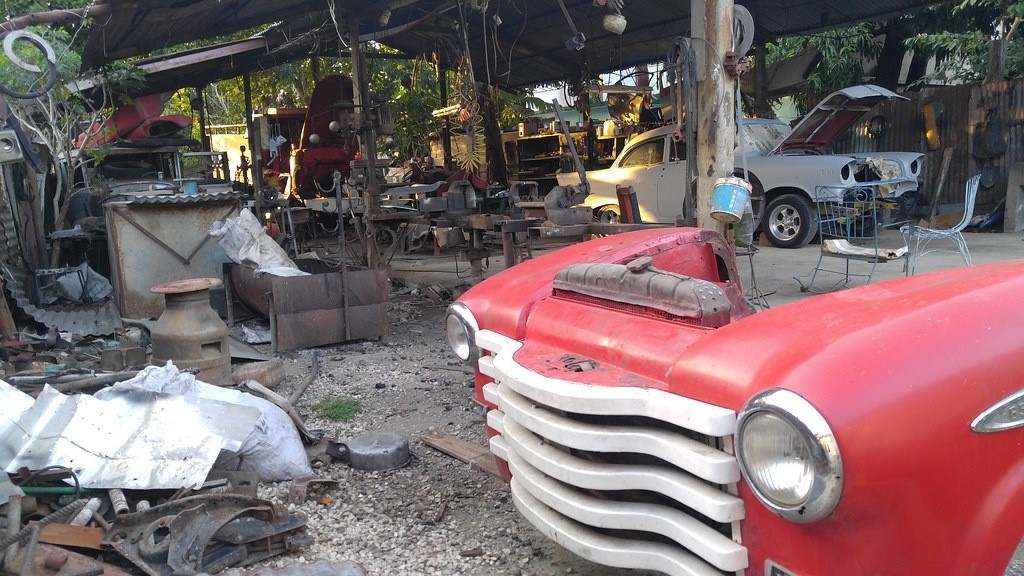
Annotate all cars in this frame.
[444,224,1024,576]
[556,84,925,250]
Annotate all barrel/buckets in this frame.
[709,177,752,224]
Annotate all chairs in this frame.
[900,173,982,276]
[795,186,912,296]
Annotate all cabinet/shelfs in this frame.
[503,131,589,197]
[595,134,629,167]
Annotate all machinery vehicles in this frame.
[259,78,362,202]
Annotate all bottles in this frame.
[603,120,614,137]
